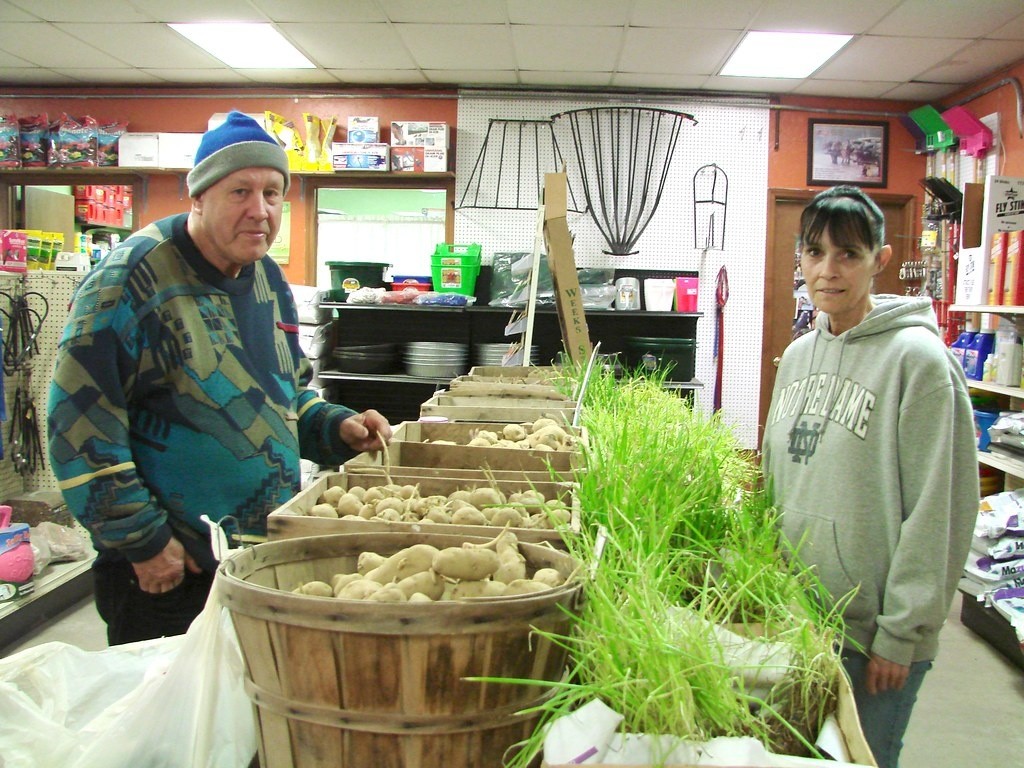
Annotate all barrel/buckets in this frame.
[325,261,393,301]
[214,532,591,768]
[975,410,999,453]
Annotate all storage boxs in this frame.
[158,133,204,168]
[391,121,450,149]
[119,132,158,166]
[332,142,390,171]
[347,116,381,143]
[391,147,449,172]
[208,113,266,132]
[265,366,590,550]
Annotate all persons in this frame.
[857,140,866,167]
[760,185,980,768]
[840,146,849,164]
[48,109,393,647]
[861,140,881,177]
[825,135,843,164]
[843,139,855,165]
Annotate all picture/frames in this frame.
[806,117,889,188]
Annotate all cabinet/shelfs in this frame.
[316,302,704,424]
[946,303,1024,479]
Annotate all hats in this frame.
[187,112,290,198]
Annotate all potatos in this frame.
[287,417,579,603]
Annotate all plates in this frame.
[334,342,538,377]
